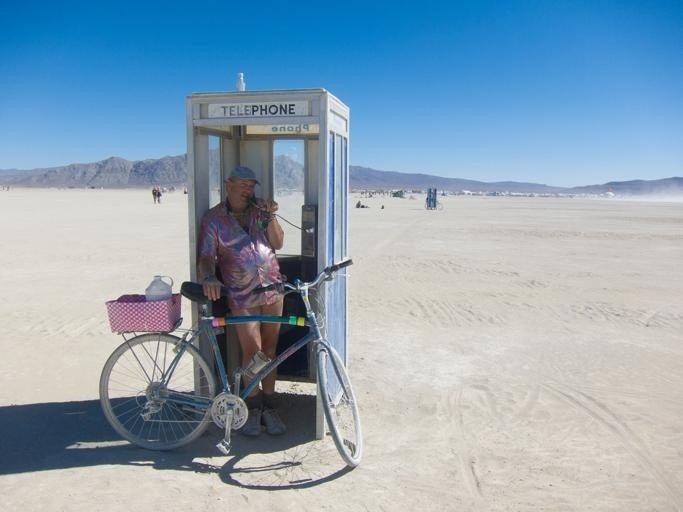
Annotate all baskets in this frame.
[106,294,181,332]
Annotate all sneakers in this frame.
[243,404,263,436]
[262,407,287,435]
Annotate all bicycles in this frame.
[99,259,361,469]
[425,200,443,209]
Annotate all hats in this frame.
[231,167,261,186]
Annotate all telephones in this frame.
[250,192,267,209]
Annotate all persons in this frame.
[196,165,288,437]
[151,186,156,204]
[156,186,162,204]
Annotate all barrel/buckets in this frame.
[145,274,174,301]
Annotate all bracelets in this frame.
[200,273,218,282]
